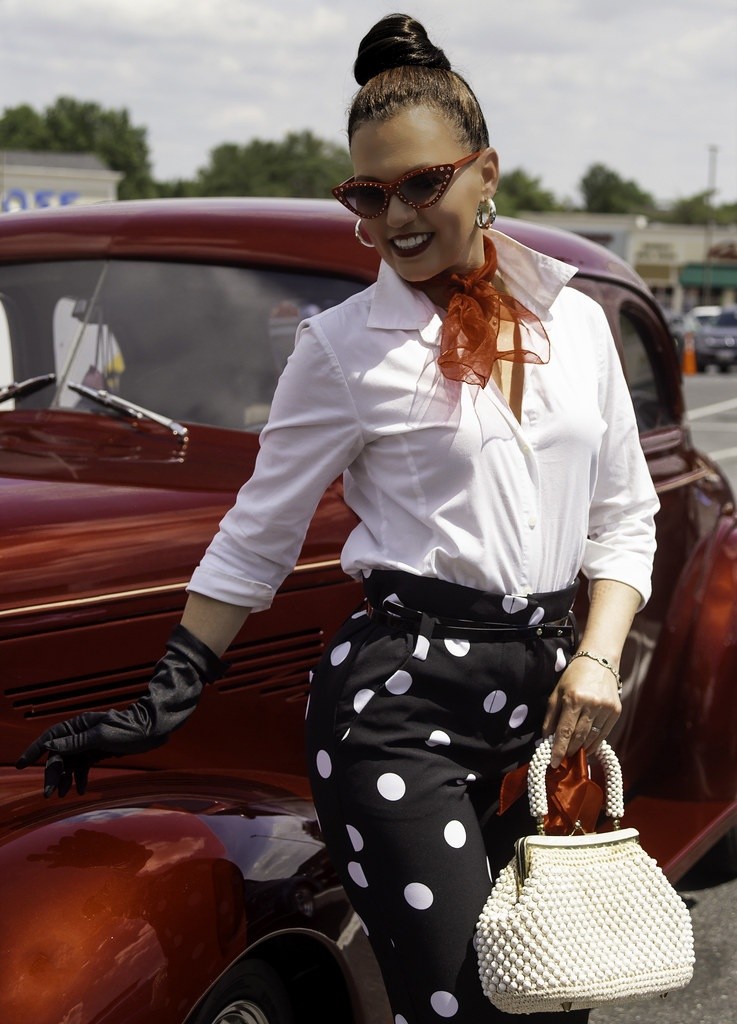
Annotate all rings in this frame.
[592,726,600,733]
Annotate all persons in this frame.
[15,14,661,1024]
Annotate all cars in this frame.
[681,305,737,369]
[1,197,737,1021]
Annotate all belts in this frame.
[362,593,577,639]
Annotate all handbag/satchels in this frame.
[475,729,696,1014]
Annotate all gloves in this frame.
[14,623,224,801]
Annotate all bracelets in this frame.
[569,651,623,694]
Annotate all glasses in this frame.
[330,149,491,220]
